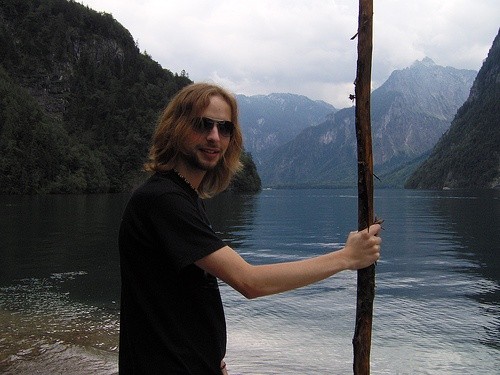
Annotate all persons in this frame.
[117,83,384,375]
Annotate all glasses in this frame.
[192,117,235,137]
